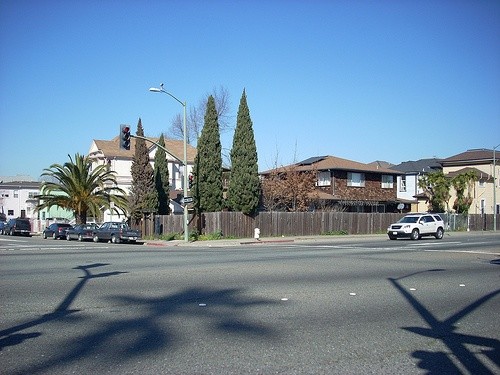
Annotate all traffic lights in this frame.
[120,124,131,150]
[188,174,193,189]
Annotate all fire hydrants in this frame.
[253,227,260,241]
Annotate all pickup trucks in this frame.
[92,222,142,243]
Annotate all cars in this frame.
[0,220,7,234]
[42,223,73,240]
[65,223,100,241]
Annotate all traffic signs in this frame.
[183,197,193,203]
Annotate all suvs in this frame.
[386,212,445,240]
[2,217,32,236]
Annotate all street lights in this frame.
[148,87,191,243]
